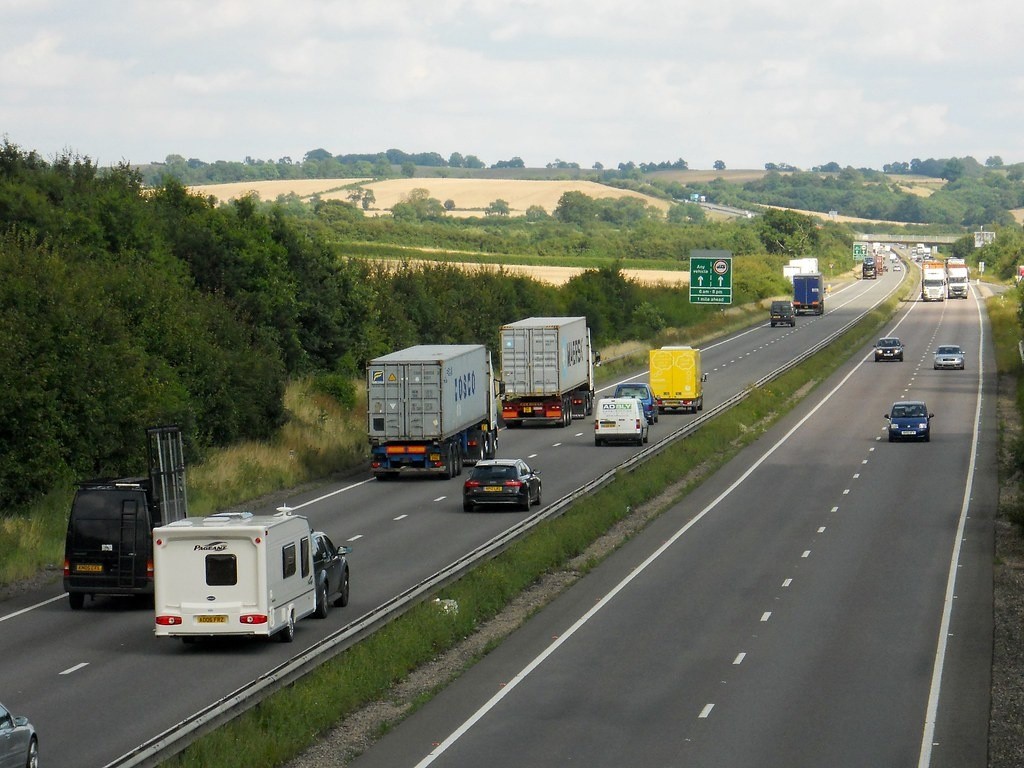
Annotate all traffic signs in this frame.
[689,257,733,305]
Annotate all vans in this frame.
[770,301,795,327]
[595,395,650,447]
[61,477,160,609]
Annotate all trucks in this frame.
[792,272,826,316]
[945,257,969,299]
[875,256,882,275]
[649,346,707,414]
[916,244,924,255]
[153,511,317,644]
[498,315,602,429]
[863,260,877,280]
[365,343,507,482]
[921,260,946,302]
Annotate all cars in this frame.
[879,243,936,272]
[932,345,966,370]
[462,459,543,512]
[311,530,351,617]
[873,337,906,362]
[614,383,661,425]
[883,401,934,442]
[0,705,39,768]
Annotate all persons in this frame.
[881,340,886,347]
[895,409,904,417]
[891,340,897,347]
[912,407,922,417]
